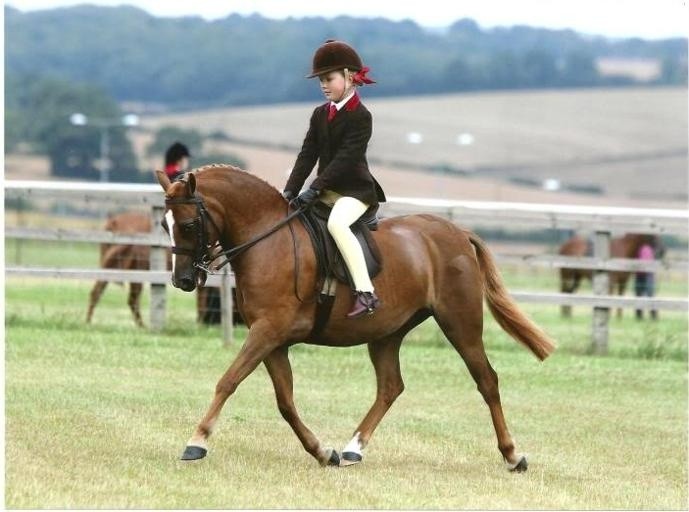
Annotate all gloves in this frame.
[282,191,294,201]
[298,190,318,208]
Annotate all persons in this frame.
[631,234,657,319]
[285,39,387,319]
[165,142,190,182]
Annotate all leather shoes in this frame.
[345,293,380,320]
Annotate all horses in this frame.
[154,162,559,474]
[558,234,666,319]
[85,207,210,328]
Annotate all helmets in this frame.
[165,143,192,164]
[304,39,363,79]
